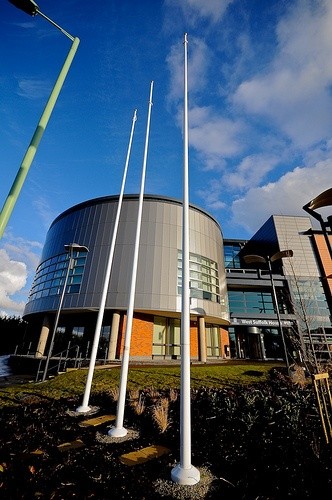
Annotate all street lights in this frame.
[0,0,79,246]
